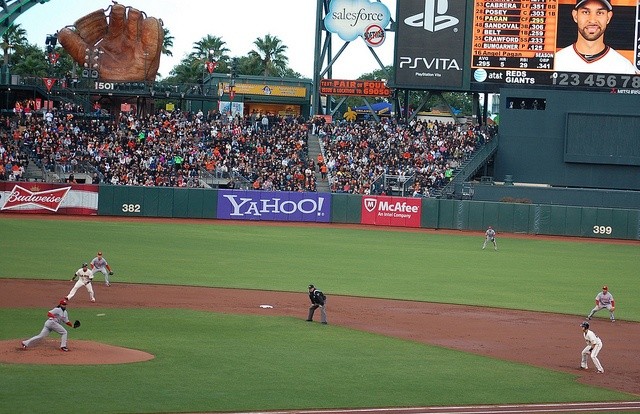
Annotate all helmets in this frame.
[580,322,589,329]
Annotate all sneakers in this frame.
[306,320,313,321]
[581,367,588,369]
[586,317,591,320]
[322,322,327,324]
[61,347,69,351]
[610,319,615,322]
[106,283,109,287]
[21,342,26,349]
[595,370,601,373]
[91,298,95,302]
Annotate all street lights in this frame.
[83,48,104,112]
[225,57,239,118]
[143,52,149,94]
[209,47,214,92]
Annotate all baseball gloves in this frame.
[109,272,113,275]
[74,320,80,328]
[610,307,615,312]
[491,239,494,242]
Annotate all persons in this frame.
[319,163,326,181]
[305,284,328,324]
[72,74,78,88]
[586,285,616,322]
[90,251,112,287]
[21,298,74,352]
[325,146,476,198]
[333,118,498,147]
[483,226,497,250]
[579,321,605,374]
[64,263,95,303]
[317,153,324,173]
[1,100,318,192]
[317,116,332,152]
[65,71,72,87]
[554,0,635,74]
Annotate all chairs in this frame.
[460,187,475,200]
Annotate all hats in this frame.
[81,263,88,268]
[574,0,613,10]
[60,300,68,305]
[308,285,314,289]
[603,286,608,290]
[98,252,102,255]
